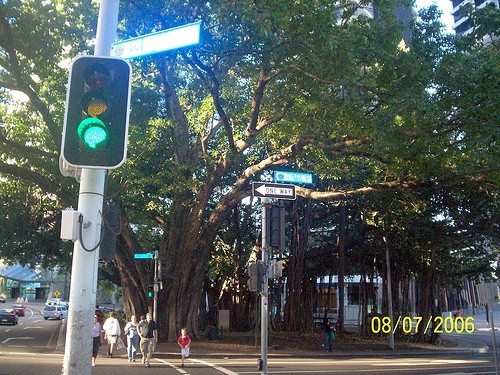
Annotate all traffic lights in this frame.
[147,286,154,299]
[248,263,262,292]
[61,57,132,170]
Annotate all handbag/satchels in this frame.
[116,337,126,350]
[331,332,335,341]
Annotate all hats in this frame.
[94,315,97,317]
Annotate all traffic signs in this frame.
[251,180,296,200]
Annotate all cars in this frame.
[11,304,26,316]
[96,305,113,312]
[0,295,6,303]
[0,307,19,325]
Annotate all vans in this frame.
[43,306,68,320]
[46,301,68,306]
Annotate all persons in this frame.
[177,328,191,359]
[324,319,333,353]
[137,313,161,367]
[455,308,461,322]
[91,315,101,367]
[124,316,140,363]
[103,312,121,355]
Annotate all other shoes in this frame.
[142,358,146,364]
[92,364,95,367]
[128,358,132,362]
[110,353,112,358]
[181,364,184,367]
[106,352,110,358]
[145,361,150,368]
[133,359,135,362]
